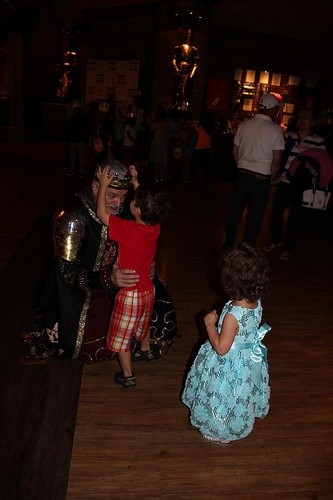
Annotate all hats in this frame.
[258,93,284,109]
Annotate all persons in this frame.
[177,242,273,447]
[9,158,178,365]
[92,162,161,390]
[319,112,333,245]
[273,108,327,263]
[218,93,286,253]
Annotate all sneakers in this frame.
[114,371,137,389]
[131,349,155,363]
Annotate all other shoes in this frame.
[263,240,281,251]
[279,252,289,260]
